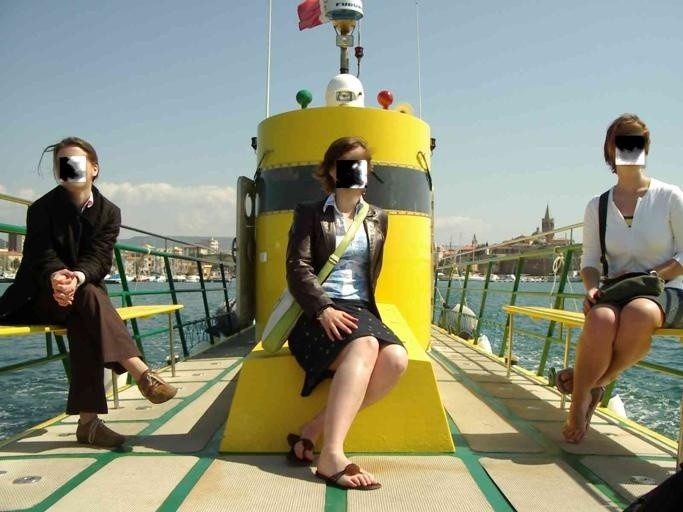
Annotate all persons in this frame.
[555,113,682,444]
[0,136,178,451]
[285,134,409,491]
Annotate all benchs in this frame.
[0,304,184,409]
[502,304,683,474]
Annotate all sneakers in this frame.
[138,370,177,405]
[75,419,124,449]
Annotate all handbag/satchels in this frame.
[589,272,664,305]
[260,289,304,354]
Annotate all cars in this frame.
[103,271,199,284]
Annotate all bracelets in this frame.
[317,306,333,321]
[647,267,657,277]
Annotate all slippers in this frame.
[285,435,315,466]
[315,464,381,491]
[563,386,602,442]
[556,368,573,395]
